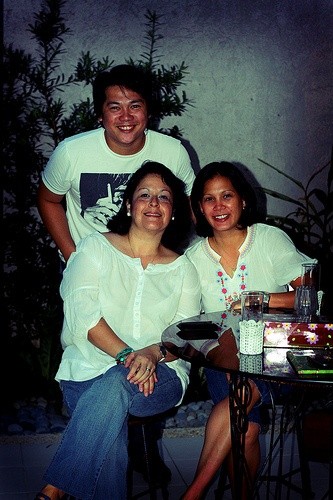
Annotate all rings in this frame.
[145,367,151,371]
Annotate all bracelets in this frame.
[114,347,136,363]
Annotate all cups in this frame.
[242,291,265,323]
[301,264,321,291]
[294,286,318,323]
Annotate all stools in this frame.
[128,415,168,500]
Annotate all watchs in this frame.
[153,343,167,363]
[262,291,271,307]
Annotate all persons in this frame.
[37,64,196,263]
[181,161,317,500]
[33,163,202,500]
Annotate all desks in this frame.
[161,306,333,500]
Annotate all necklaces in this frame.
[127,234,159,263]
